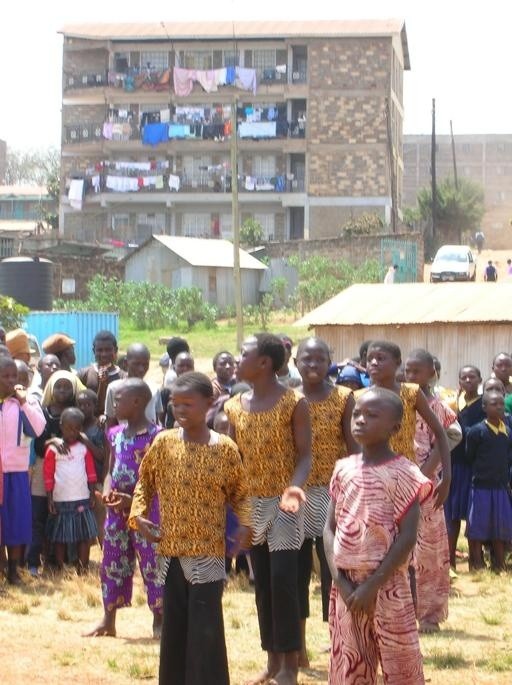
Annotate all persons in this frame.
[475,229,487,255]
[484,260,498,282]
[384,264,399,284]
[507,258,512,275]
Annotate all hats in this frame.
[5,329,36,358]
[42,334,76,354]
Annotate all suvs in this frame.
[429,244,477,283]
